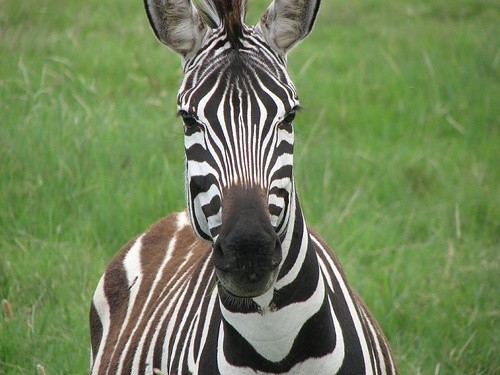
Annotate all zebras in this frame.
[90,0,397,375]
